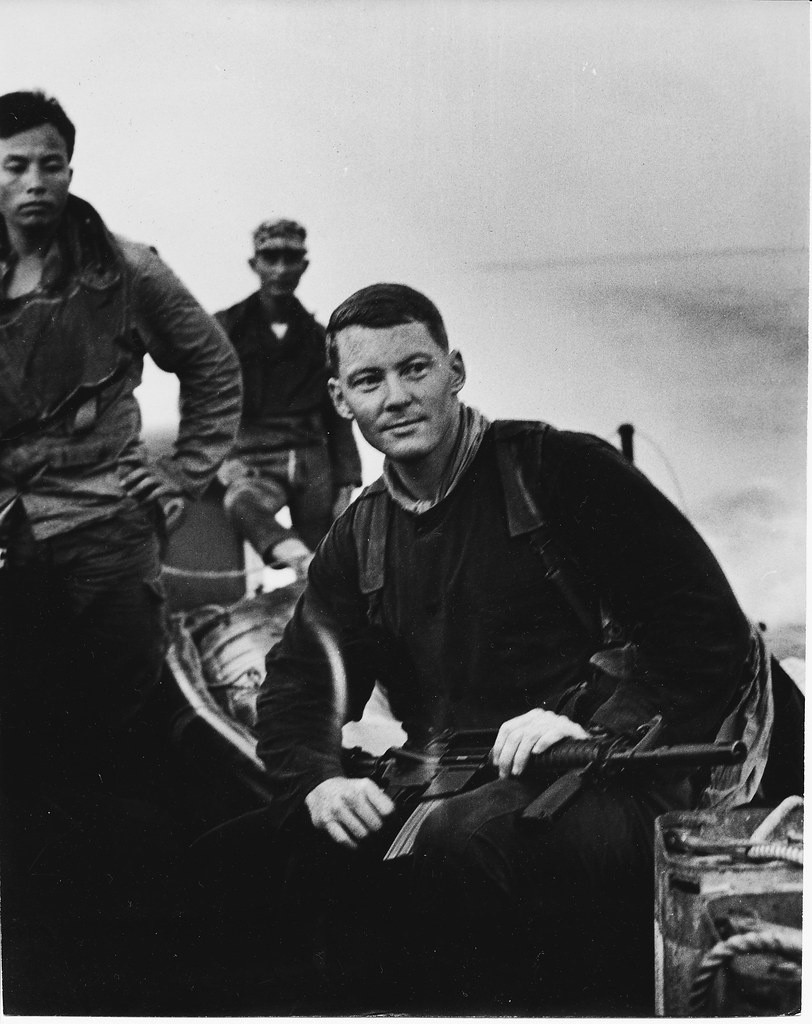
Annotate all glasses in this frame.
[258,247,305,265]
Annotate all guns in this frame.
[337,682,744,864]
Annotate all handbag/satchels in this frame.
[521,627,794,871]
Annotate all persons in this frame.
[256,282,774,886]
[0,89,363,727]
